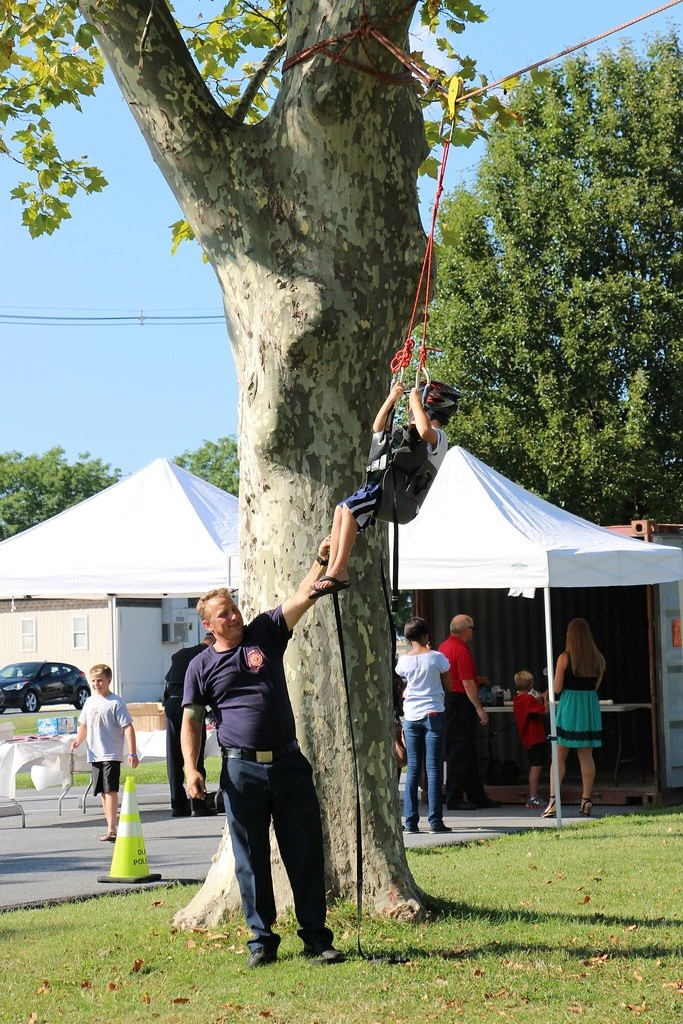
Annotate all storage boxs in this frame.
[37,715,77,736]
[124,702,165,730]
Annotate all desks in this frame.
[472,703,653,788]
[0,733,93,827]
[78,728,226,814]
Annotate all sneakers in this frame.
[526,796,548,808]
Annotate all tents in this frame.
[1,456,239,698]
[381,443,683,827]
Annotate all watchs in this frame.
[316,555,329,566]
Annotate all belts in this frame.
[222,739,300,763]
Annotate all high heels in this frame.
[578,797,592,818]
[542,796,557,818]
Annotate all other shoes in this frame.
[467,799,502,808]
[447,799,475,811]
[248,949,277,970]
[405,827,419,833]
[172,804,218,818]
[303,944,345,965]
[430,826,453,833]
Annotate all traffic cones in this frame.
[97,776,163,884]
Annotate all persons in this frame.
[512,668,550,809]
[541,618,605,819]
[395,617,451,834]
[163,632,217,818]
[309,378,463,603]
[395,638,408,832]
[437,614,503,810]
[71,664,140,842]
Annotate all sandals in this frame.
[101,832,117,841]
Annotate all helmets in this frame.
[403,380,461,426]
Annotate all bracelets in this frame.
[128,753,137,757]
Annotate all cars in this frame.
[0,661,92,714]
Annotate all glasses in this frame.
[468,626,474,631]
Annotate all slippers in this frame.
[312,574,351,590]
[308,588,334,599]
